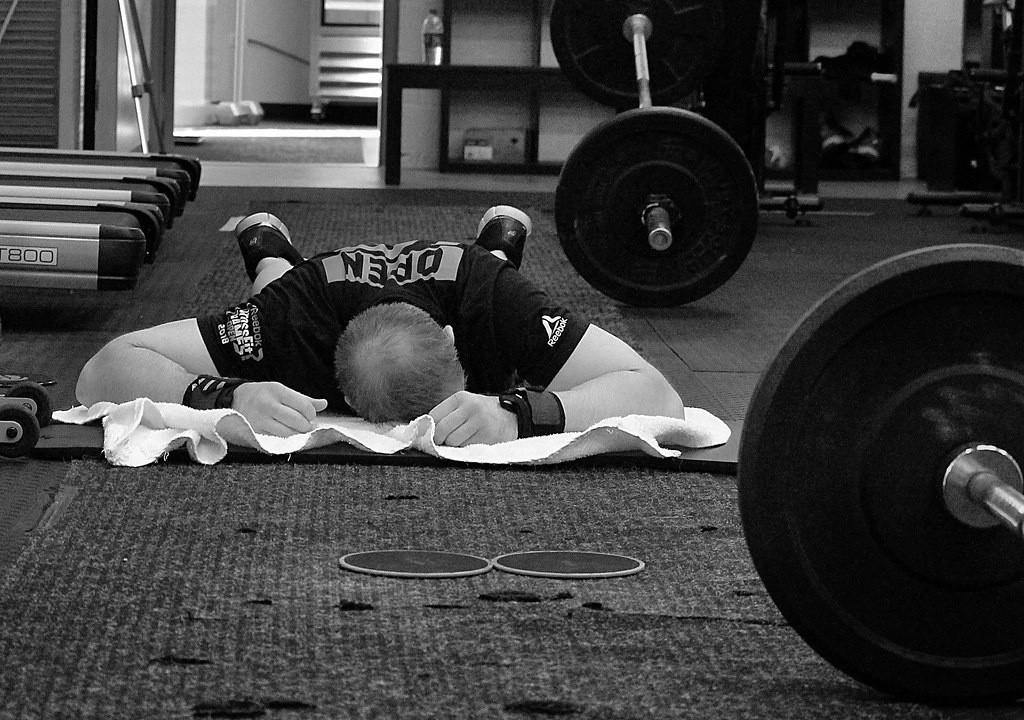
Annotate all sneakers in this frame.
[235,212,305,285]
[474,205,532,272]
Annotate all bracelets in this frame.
[499,386,566,441]
[181,372,250,411]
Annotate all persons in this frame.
[76,204,687,447]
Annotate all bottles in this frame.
[421,9,444,65]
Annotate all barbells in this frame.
[544,0,767,314]
[724,228,1024,715]
